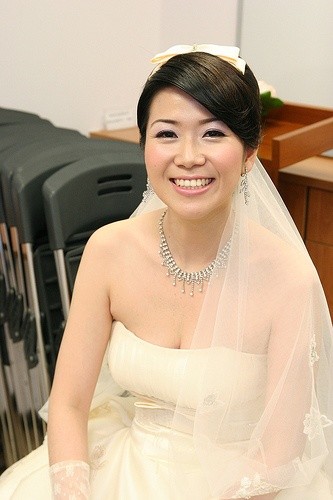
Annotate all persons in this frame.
[1,43,333,499]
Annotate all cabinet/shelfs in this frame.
[88,102,333,325]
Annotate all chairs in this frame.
[0,108,147,469]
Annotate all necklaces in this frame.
[157,206,239,300]
